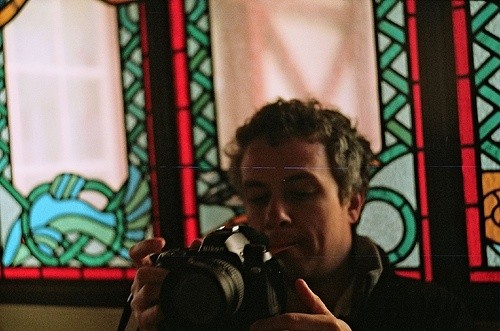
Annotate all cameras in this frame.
[150,226,290,331]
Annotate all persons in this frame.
[130,97,454,331]
